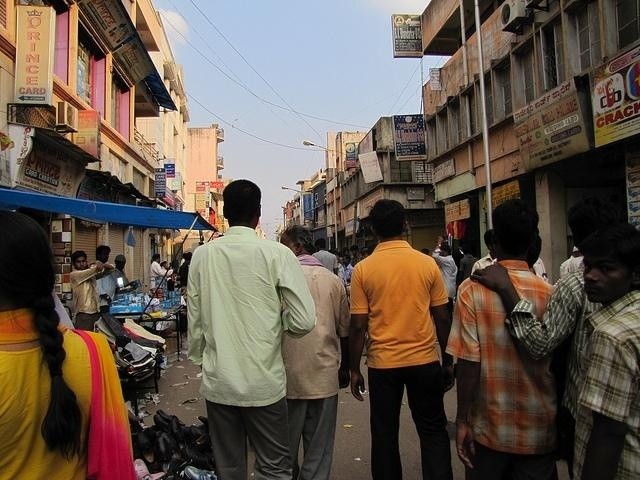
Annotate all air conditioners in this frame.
[56,102,78,132]
[498,0,526,29]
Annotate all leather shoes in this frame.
[130,409,219,480]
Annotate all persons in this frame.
[69,245,129,330]
[1,208,134,479]
[347,199,456,480]
[277,225,370,479]
[187,179,316,478]
[150,252,192,293]
[419,234,479,310]
[446,196,639,478]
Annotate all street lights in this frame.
[282,186,304,228]
[304,141,338,249]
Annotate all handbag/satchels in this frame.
[74,328,136,480]
